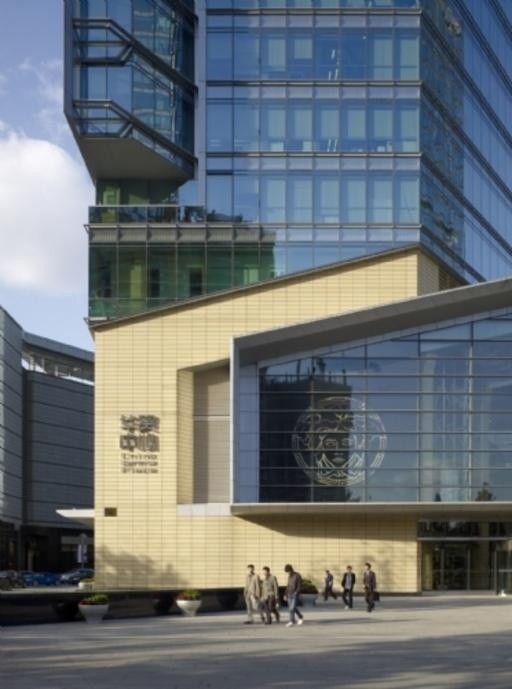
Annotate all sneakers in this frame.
[344,604,353,610]
[367,603,375,612]
[244,615,303,627]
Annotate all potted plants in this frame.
[77,578,95,591]
[297,578,319,607]
[78,594,111,623]
[174,591,201,616]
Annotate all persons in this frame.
[243,564,265,623]
[340,566,356,609]
[261,567,279,624]
[475,482,493,502]
[283,564,304,626]
[363,564,375,612]
[325,570,337,600]
